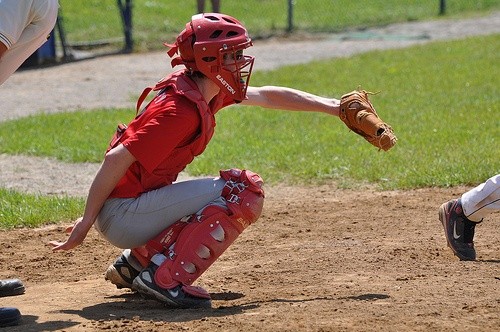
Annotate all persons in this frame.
[48,11,398,307]
[0,0,61,326]
[438,173,500,262]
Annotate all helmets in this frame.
[164,12,252,101]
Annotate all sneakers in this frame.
[105,249,152,296]
[133,254,212,309]
[437,199,483,261]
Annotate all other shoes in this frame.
[0,278,26,297]
[0,308,22,327]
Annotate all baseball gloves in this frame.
[337,85,400,152]
